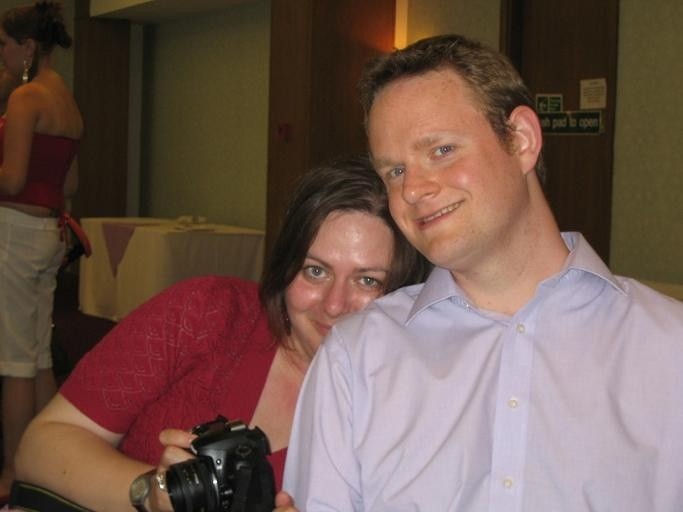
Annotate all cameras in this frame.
[165,414,275,512]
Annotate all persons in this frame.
[13,156,436,512]
[0,0,85,506]
[281,34,683,512]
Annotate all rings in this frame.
[155,473,166,490]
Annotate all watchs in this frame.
[129,469,157,512]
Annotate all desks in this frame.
[77,216,265,330]
